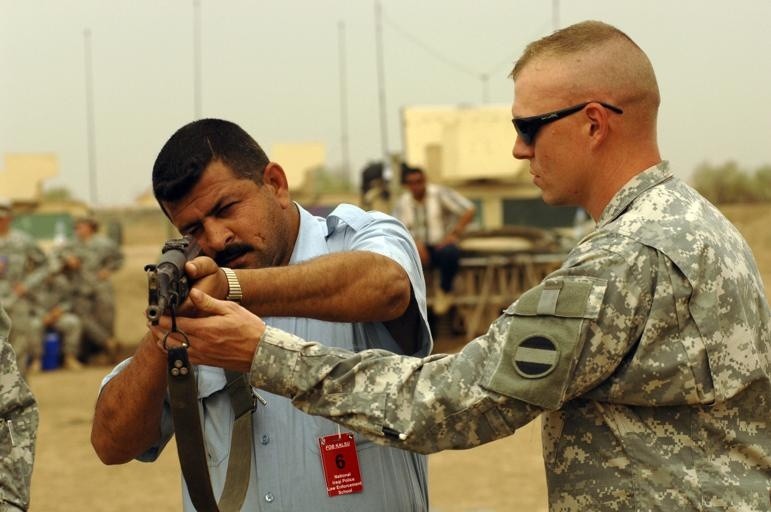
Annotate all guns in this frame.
[144,233,204,326]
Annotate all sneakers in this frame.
[433,289,451,313]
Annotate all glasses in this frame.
[513,102,623,146]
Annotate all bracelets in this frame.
[221,267,242,305]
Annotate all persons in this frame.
[392,169,476,315]
[0,304,39,512]
[91,119,434,512]
[0,219,125,370]
[144,21,770,512]
